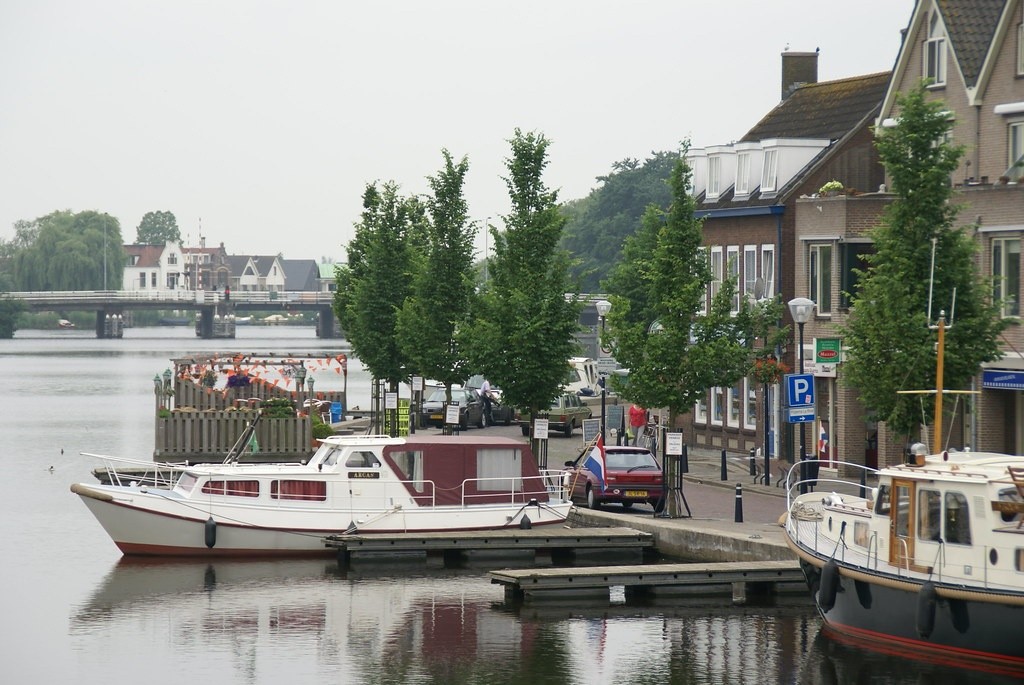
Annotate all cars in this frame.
[422,383,512,428]
[514,390,593,438]
[558,446,668,512]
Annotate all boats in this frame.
[778,238,1024,685]
[72,411,574,558]
[59,319,76,329]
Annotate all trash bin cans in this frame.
[806,455,819,486]
[331,401,342,423]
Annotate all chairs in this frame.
[305,399,333,425]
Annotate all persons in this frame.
[629,403,648,448]
[479,380,499,426]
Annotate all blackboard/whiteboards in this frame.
[582,419,601,444]
[607,406,623,431]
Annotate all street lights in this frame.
[787,298,817,495]
[596,299,611,446]
[103,212,108,292]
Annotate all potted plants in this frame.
[819,178,844,197]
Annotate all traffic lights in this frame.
[225,286,230,300]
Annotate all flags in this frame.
[249,432,259,454]
[582,433,609,494]
[818,421,829,453]
